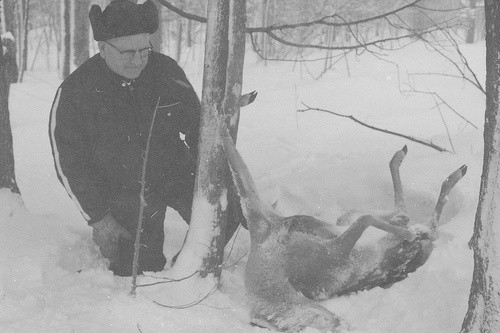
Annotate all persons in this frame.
[46,0,245,280]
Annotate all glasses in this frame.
[104,39,153,58]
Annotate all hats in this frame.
[90,0,158,41]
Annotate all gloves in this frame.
[92,214,131,260]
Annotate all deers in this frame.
[219,89,469,333]
